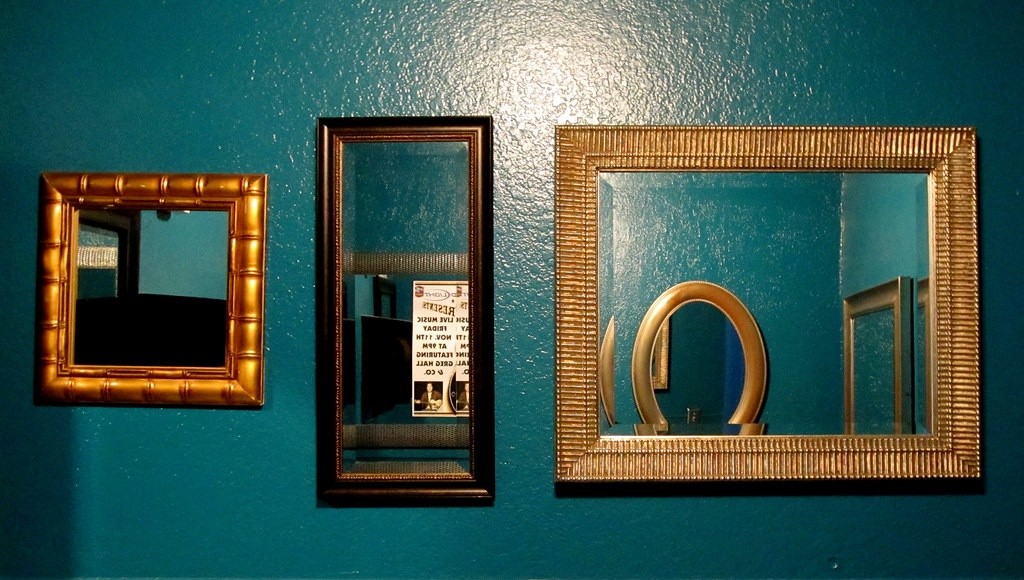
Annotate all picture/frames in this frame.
[651,317,670,391]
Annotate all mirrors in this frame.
[315,115,496,507]
[839,274,912,435]
[631,279,768,425]
[31,170,267,408]
[553,123,982,484]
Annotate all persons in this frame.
[421,383,442,411]
[458,382,469,410]
